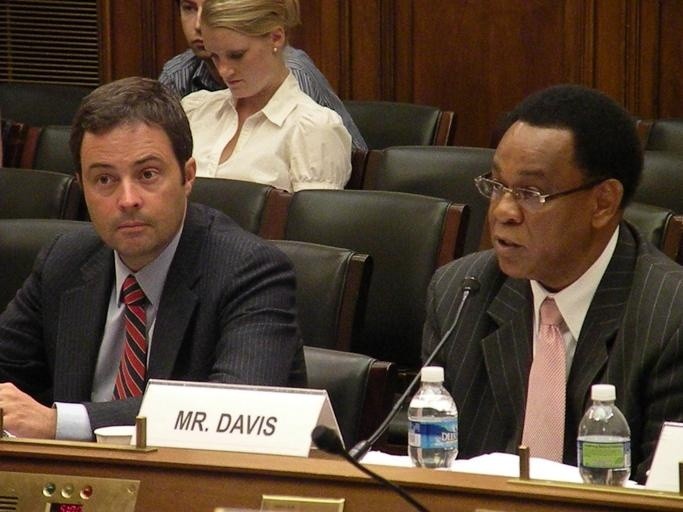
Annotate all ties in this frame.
[519,298,568,463]
[109,275,150,399]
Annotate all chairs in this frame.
[622,201,683,263]
[19,125,75,174]
[0,167,74,222]
[1,219,91,313]
[343,101,457,150]
[66,177,289,239]
[305,347,396,449]
[344,148,367,191]
[632,119,683,150]
[630,150,683,213]
[366,146,495,258]
[276,190,471,370]
[0,119,25,168]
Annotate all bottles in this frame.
[577,385,631,491]
[407,365,458,470]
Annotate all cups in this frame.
[93,425,135,445]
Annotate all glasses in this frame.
[473,169,611,216]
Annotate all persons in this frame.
[421,86,682,482]
[0,76,308,441]
[159,1,369,161]
[180,0,353,193]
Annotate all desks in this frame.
[0,433,683,512]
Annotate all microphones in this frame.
[350,275,481,462]
[310,425,432,512]
